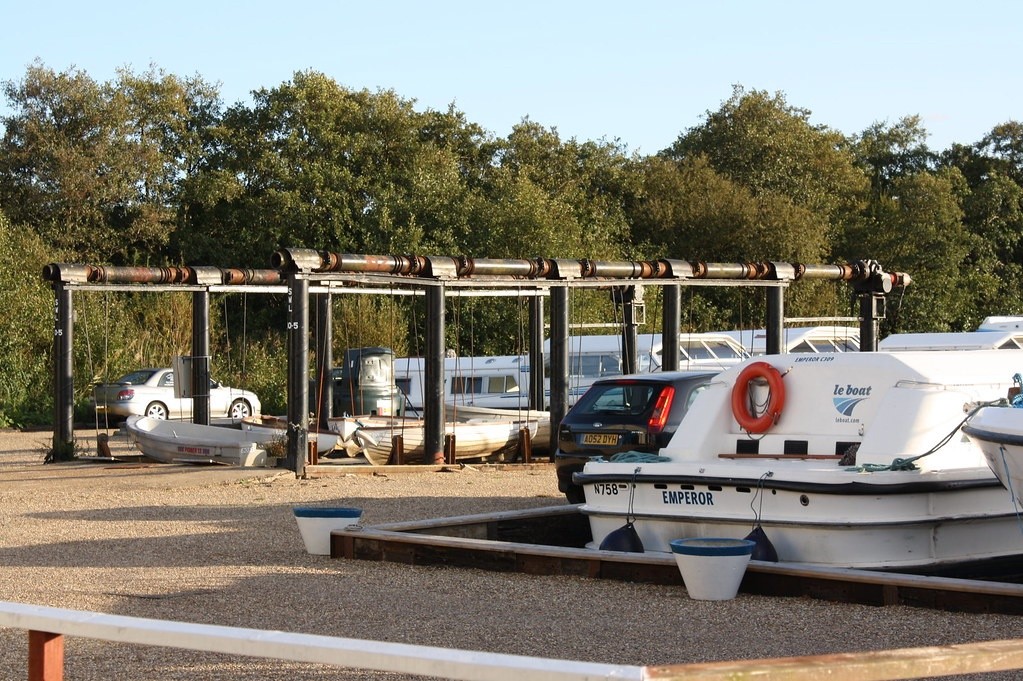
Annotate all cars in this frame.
[92,366,263,429]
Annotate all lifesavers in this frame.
[730,360,787,434]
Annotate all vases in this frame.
[292,508,364,555]
[670,536,758,601]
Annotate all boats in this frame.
[310,318,860,407]
[126,412,289,467]
[444,401,552,455]
[355,417,538,469]
[322,411,398,451]
[962,374,1023,515]
[570,347,1023,591]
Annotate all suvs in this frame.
[553,368,720,502]
